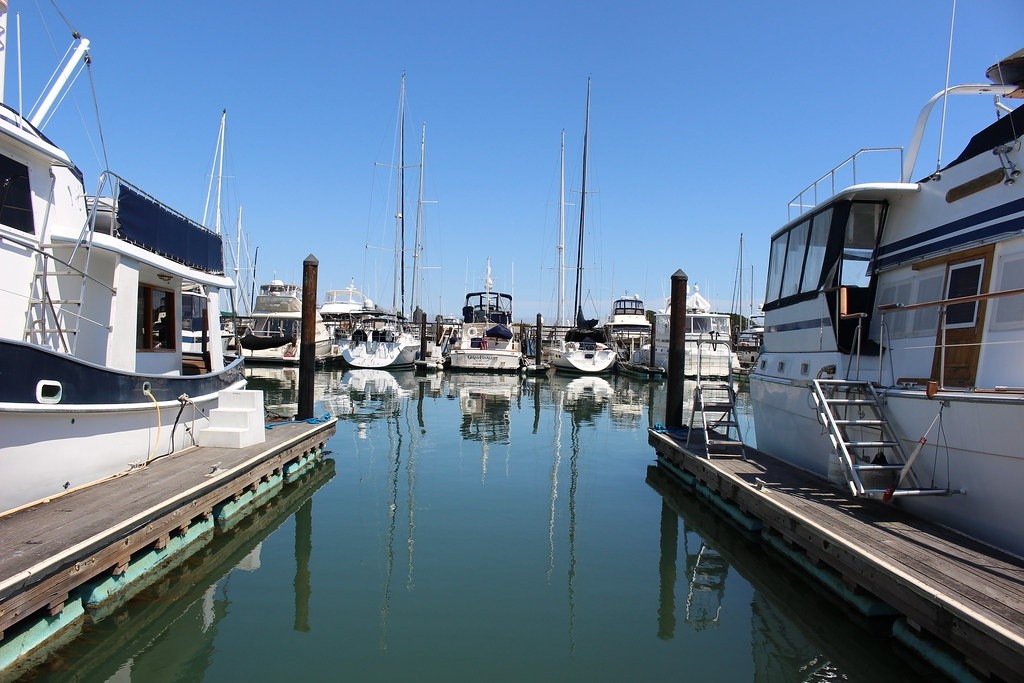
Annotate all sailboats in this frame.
[239,363,738,632]
[197,68,778,372]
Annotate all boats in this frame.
[748,45,1023,562]
[0,99,248,520]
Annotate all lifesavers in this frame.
[467,327,478,337]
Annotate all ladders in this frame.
[686,341,747,461]
[682,520,730,624]
